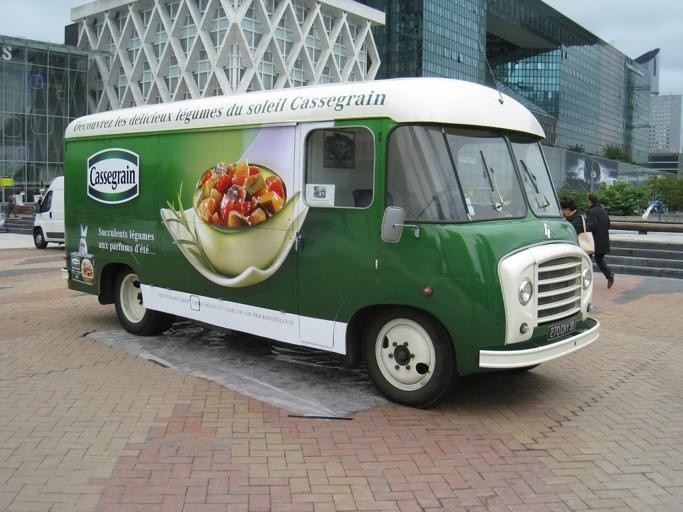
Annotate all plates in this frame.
[158,207,311,287]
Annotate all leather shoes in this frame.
[606,272,614,288]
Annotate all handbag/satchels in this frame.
[576,212,596,255]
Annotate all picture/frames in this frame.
[322,129,356,169]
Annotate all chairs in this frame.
[353,189,394,211]
[434,190,469,221]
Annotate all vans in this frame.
[34,176,65,249]
[64,77,601,407]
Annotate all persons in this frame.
[559,197,589,238]
[580,192,615,291]
